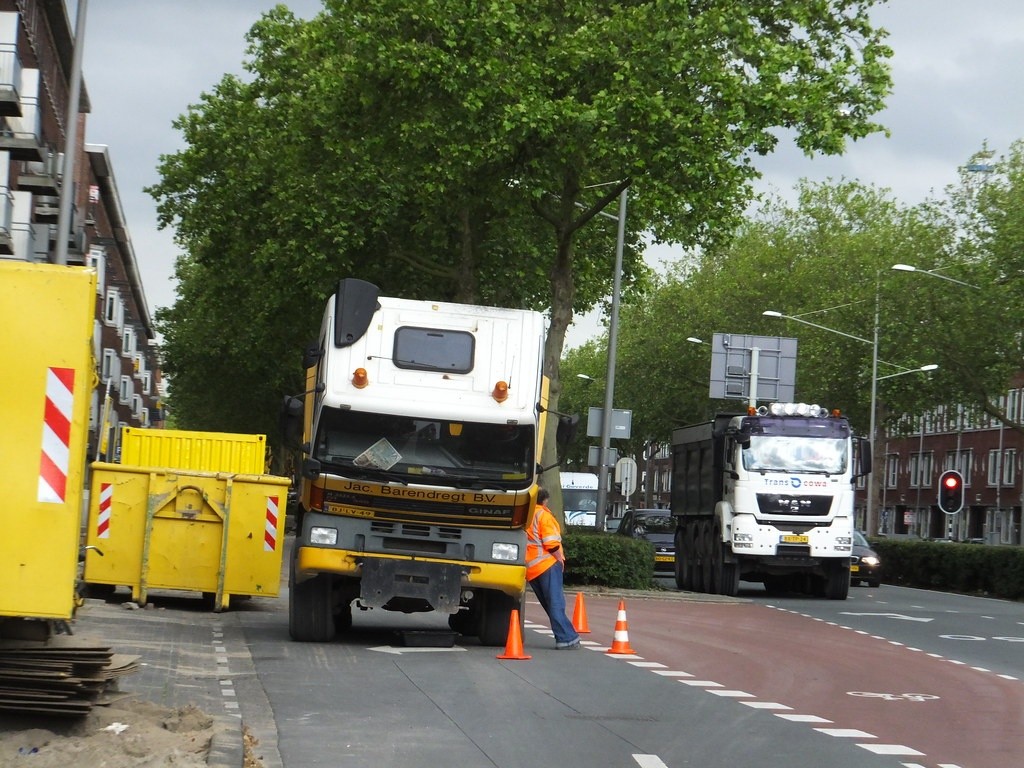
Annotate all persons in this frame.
[525,488,580,649]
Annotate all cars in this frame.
[614,508,678,572]
[850,530,881,587]
[964,537,990,546]
[923,537,955,543]
[606,517,623,533]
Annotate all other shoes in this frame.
[556,637,580,650]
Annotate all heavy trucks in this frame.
[671,403,872,601]
[282,278,580,649]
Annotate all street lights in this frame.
[763,270,939,538]
[495,175,627,531]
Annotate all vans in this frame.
[558,471,608,530]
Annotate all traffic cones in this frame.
[571,592,592,633]
[607,599,638,655]
[496,610,532,659]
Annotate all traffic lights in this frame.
[938,470,963,514]
